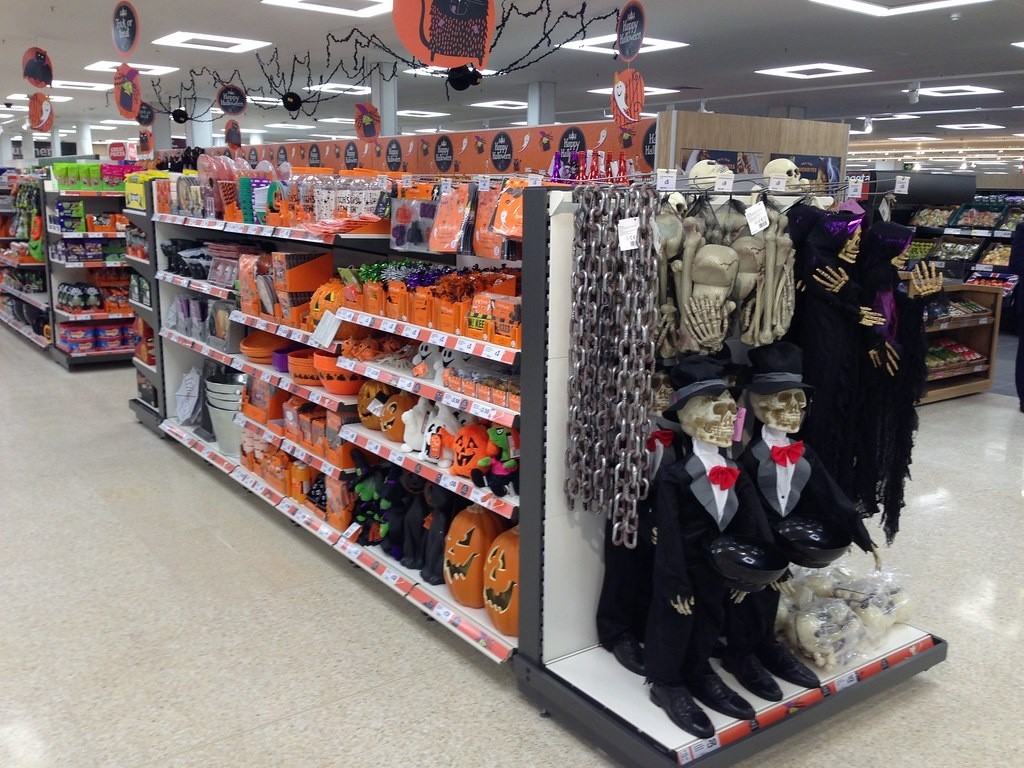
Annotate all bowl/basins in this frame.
[287,349,371,394]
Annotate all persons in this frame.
[1008,221,1024,413]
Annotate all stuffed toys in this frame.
[346,450,457,585]
[400,396,521,497]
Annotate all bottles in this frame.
[289,167,392,220]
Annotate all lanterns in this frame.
[341,334,421,361]
[357,379,421,443]
[310,272,380,340]
[443,503,519,637]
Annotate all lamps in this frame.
[907,80,921,104]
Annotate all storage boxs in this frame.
[49,172,521,533]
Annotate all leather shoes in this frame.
[686,668,756,720]
[720,653,784,702]
[759,639,821,688]
[650,681,715,739]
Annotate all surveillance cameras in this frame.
[4,103,12,108]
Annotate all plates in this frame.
[239,331,293,364]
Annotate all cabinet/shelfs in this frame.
[0,173,134,370]
[122,155,1024,768]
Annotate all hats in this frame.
[662,356,743,421]
[741,341,813,395]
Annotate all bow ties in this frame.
[646,430,673,451]
[708,465,739,491]
[771,441,803,466]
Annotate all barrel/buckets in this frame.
[205,373,249,457]
